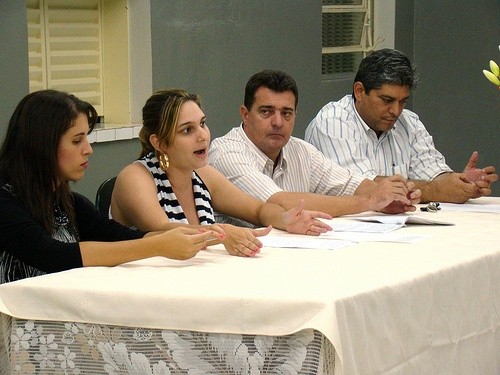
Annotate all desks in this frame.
[0,196,500,375]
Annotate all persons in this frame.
[106,89,333,256]
[303,46,498,204]
[203,68,420,232]
[0,89,228,284]
